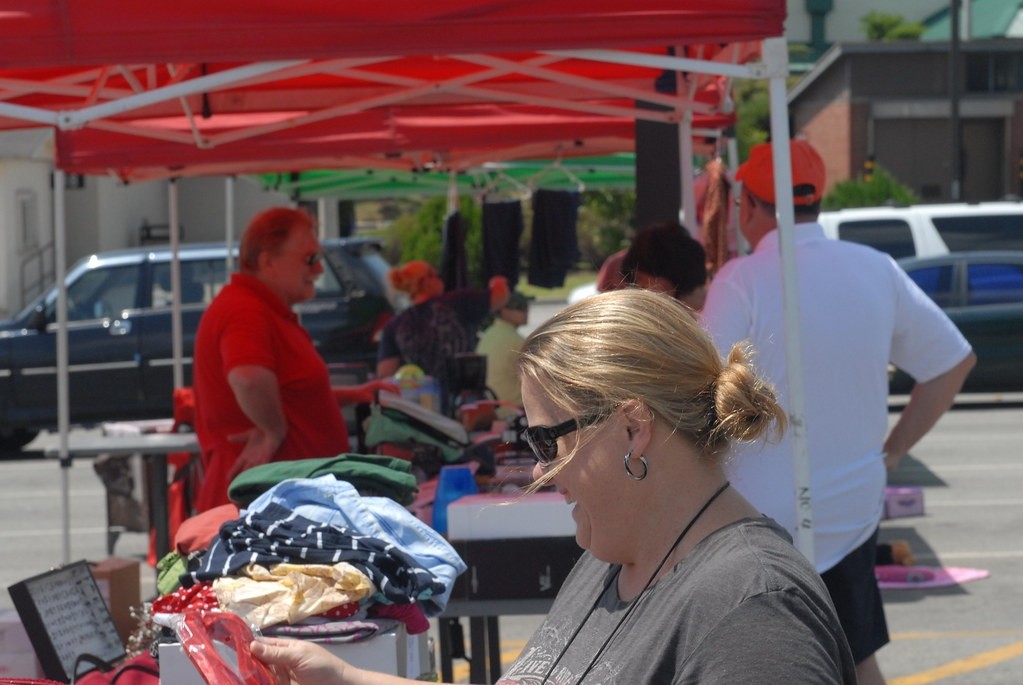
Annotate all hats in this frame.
[735,141,826,205]
[503,293,536,308]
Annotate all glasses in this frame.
[510,302,530,311]
[271,247,322,268]
[524,402,623,462]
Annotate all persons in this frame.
[472,286,543,421]
[597,224,689,291]
[701,140,976,684]
[377,259,476,427]
[191,205,404,517]
[622,229,711,316]
[248,287,856,685]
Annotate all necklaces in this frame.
[534,477,728,684]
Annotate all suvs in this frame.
[1,234,413,459]
[814,200,1022,266]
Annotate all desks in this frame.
[435,600,554,685]
[26,432,199,601]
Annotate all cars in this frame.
[885,250,1023,394]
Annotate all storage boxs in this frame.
[158,621,432,685]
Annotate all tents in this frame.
[0,2,820,683]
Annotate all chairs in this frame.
[189,281,205,303]
